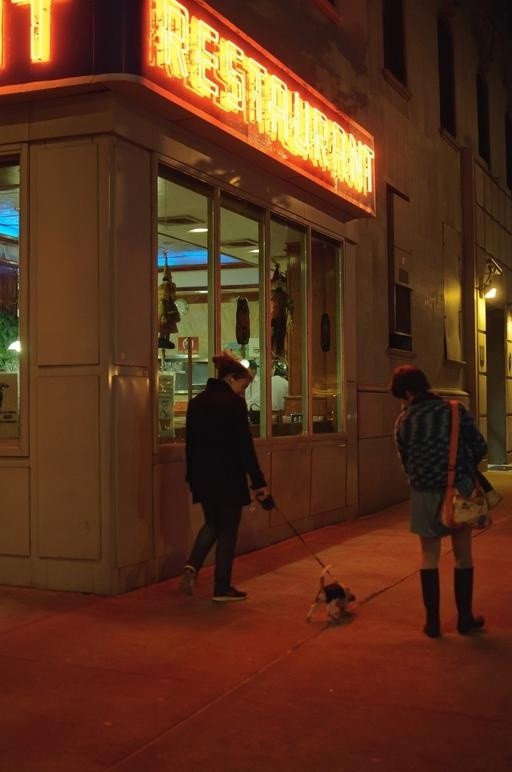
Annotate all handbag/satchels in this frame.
[440,485,493,530]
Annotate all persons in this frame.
[243,359,260,410]
[176,346,271,603]
[272,362,290,412]
[387,363,490,640]
[269,264,296,359]
[155,254,181,351]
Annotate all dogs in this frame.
[306,563,355,627]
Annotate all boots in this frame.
[454,566,485,634]
[420,567,440,638]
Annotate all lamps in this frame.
[476,258,504,301]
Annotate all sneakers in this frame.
[214,582,247,603]
[180,562,197,598]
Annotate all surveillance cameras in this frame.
[485,256,503,278]
